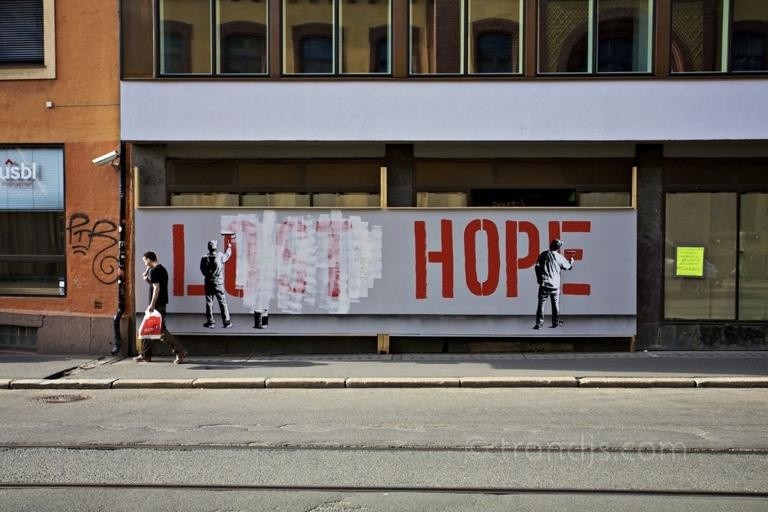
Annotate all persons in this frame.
[533,238,574,329]
[132,252,188,363]
[199,238,232,328]
[726,230,751,281]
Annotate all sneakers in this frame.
[132,353,152,362]
[203,321,216,329]
[173,348,188,365]
[552,321,564,329]
[224,321,233,329]
[534,321,544,330]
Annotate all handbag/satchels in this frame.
[138,307,164,340]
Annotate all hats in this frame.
[208,238,217,249]
[549,238,564,251]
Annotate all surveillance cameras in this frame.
[92,150,120,166]
